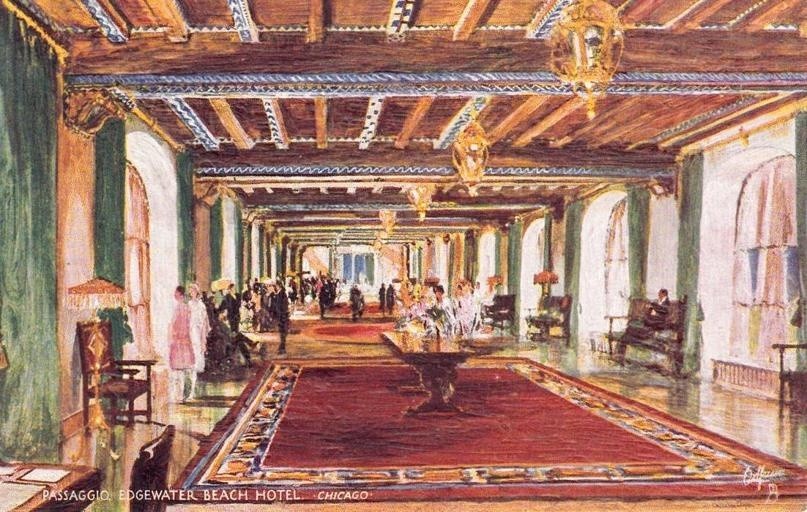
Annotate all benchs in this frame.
[769,343,806,418]
[527,294,571,343]
[604,294,704,379]
[480,294,515,328]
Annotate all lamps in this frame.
[404,185,436,221]
[545,1,624,121]
[446,100,493,199]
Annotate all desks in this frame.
[379,330,480,423]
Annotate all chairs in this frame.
[77,321,154,428]
[124,426,173,512]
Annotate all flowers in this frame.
[486,275,502,291]
[535,270,558,284]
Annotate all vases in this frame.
[541,283,550,294]
[494,282,502,293]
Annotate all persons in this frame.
[608,289,673,364]
[166,269,399,404]
[434,280,481,335]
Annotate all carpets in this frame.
[170,359,807,499]
[305,323,392,345]
